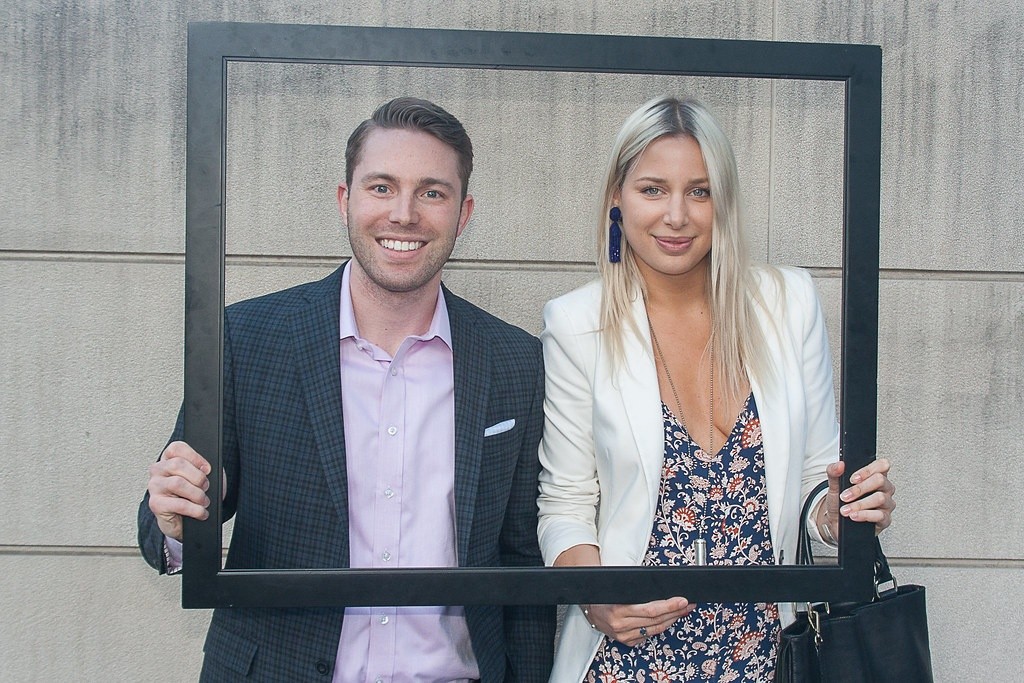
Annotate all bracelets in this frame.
[582,605,601,632]
[821,503,838,545]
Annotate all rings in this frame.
[639,626,646,638]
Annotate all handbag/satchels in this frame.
[774,479,934,683]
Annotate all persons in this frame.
[536,95,897,683]
[137,98,558,683]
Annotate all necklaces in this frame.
[647,313,714,567]
